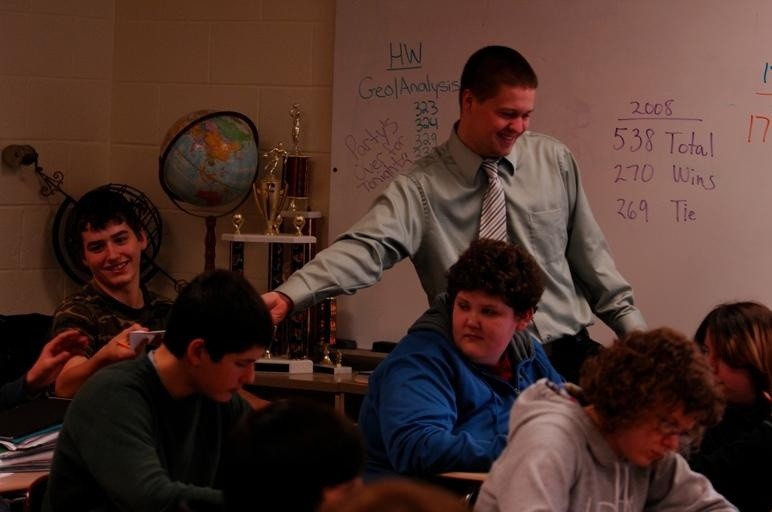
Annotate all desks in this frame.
[254,353,371,415]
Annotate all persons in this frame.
[684,302,768,512]
[42,269,272,512]
[0,329,88,422]
[261,45,649,381]
[473,328,741,512]
[229,398,471,512]
[54,191,173,397]
[359,239,585,492]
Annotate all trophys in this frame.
[331,349,344,367]
[319,346,335,367]
[281,101,310,213]
[264,350,270,360]
[231,212,244,236]
[253,143,287,237]
[293,215,306,235]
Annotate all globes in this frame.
[159,111,263,271]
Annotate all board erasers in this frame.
[372,340,400,355]
[328,339,359,349]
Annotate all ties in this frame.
[478,160,507,243]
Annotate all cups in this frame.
[126,331,167,359]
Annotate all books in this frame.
[0,423,62,472]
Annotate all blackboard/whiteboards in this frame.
[327,1,772,349]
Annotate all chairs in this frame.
[0,313,71,439]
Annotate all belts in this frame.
[542,330,589,358]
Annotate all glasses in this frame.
[656,413,697,445]
